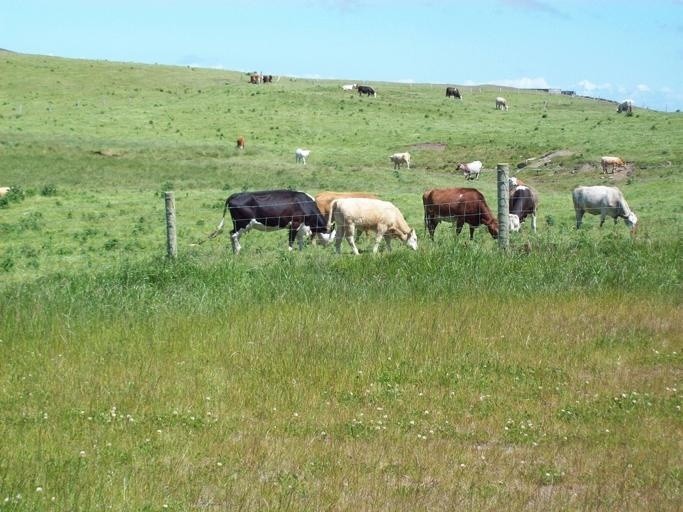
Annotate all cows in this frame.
[455,160,483,181]
[600,157,628,175]
[295,148,311,166]
[237,136,245,150]
[209,189,419,255]
[495,97,509,112]
[342,83,376,98]
[422,187,499,242]
[446,87,463,99]
[572,185,639,235]
[618,99,634,113]
[388,152,411,169]
[508,176,538,234]
